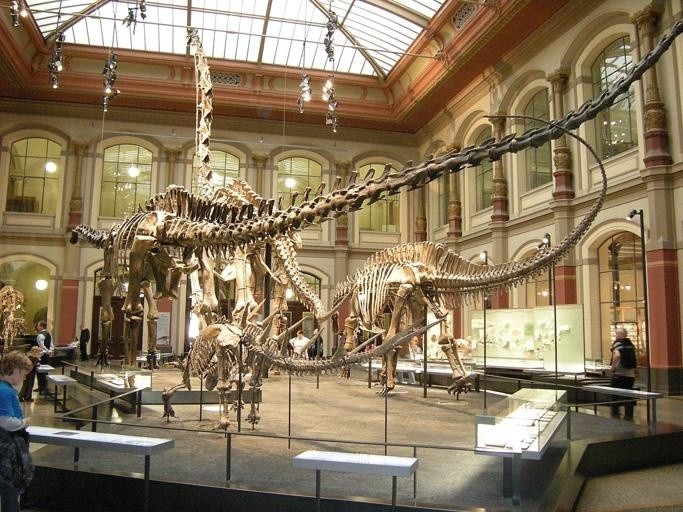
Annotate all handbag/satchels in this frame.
[0,428,34,496]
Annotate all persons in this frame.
[604,327,637,422]
[70,335,81,361]
[306,327,322,360]
[0,349,36,511]
[429,334,440,359]
[14,345,42,403]
[79,324,90,361]
[287,330,310,361]
[363,343,376,352]
[31,320,54,392]
[393,321,416,385]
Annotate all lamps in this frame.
[297,0,312,115]
[10,0,20,27]
[101,2,120,113]
[322,0,339,133]
[48,0,65,90]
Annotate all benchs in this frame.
[360,357,612,415]
[293,450,420,512]
[36,364,55,395]
[584,386,662,423]
[47,375,78,413]
[24,426,176,502]
